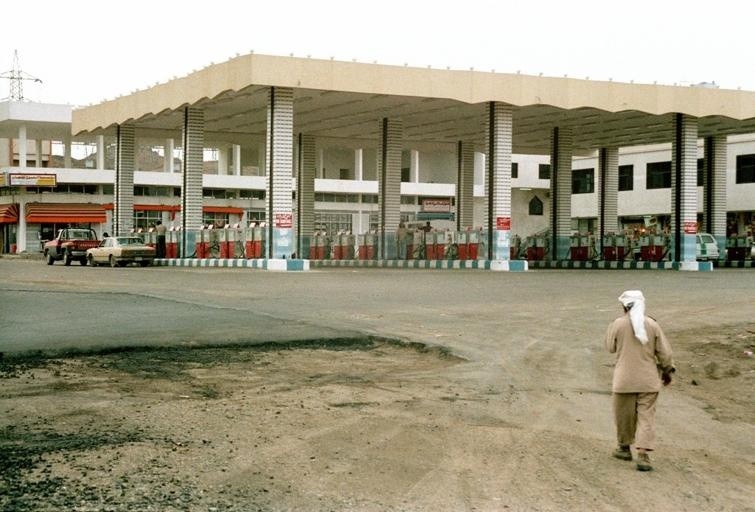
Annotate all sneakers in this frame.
[635,453,652,470]
[612,449,631,461]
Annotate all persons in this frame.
[398,223,413,260]
[102,232,109,238]
[155,220,166,259]
[417,222,434,232]
[607,290,676,470]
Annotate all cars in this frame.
[695,232,720,267]
[84,235,159,269]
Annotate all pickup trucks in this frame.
[41,225,104,266]
